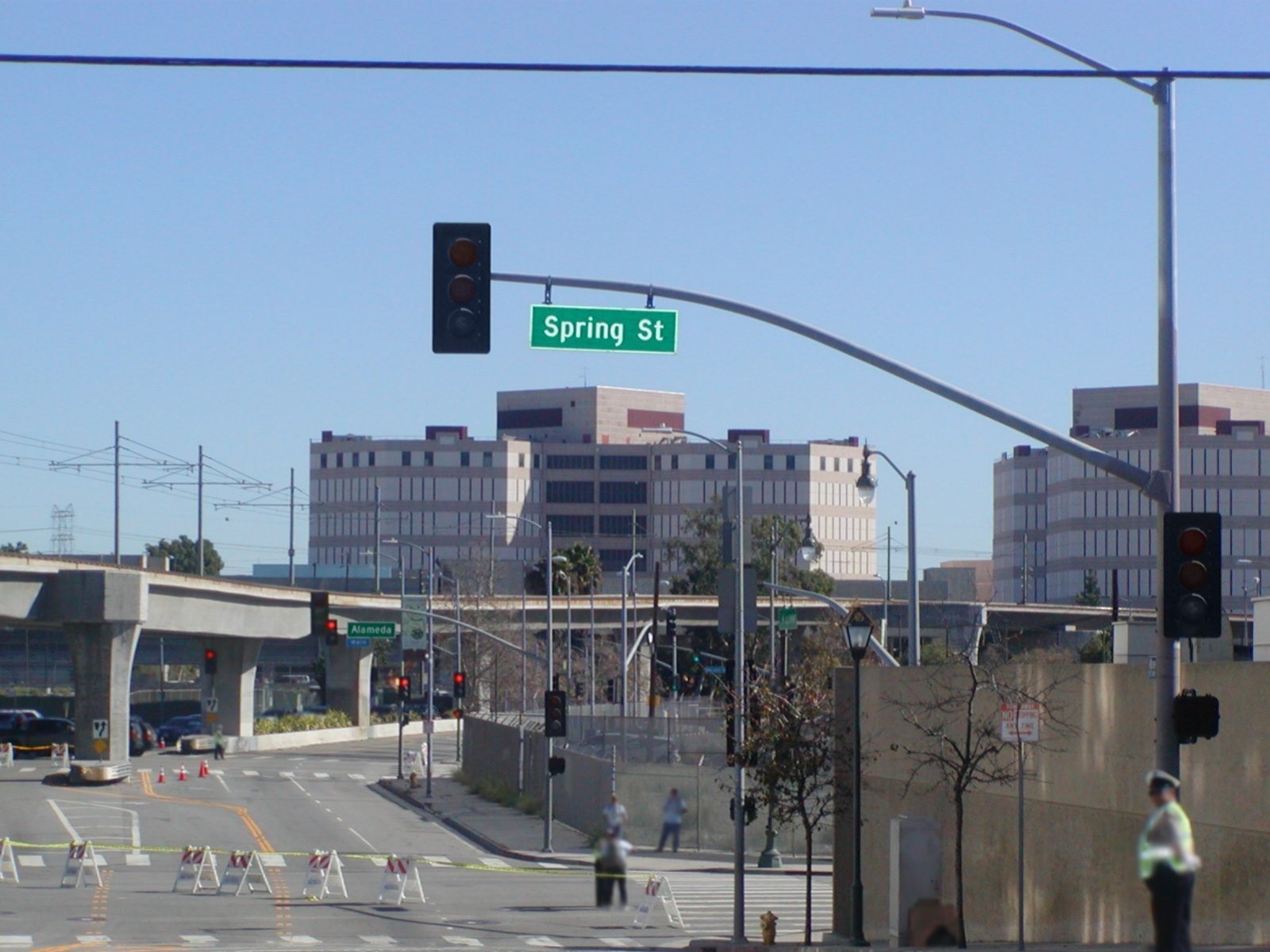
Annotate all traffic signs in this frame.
[528,305,680,355]
[348,622,397,639]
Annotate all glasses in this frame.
[1149,789,1167,796]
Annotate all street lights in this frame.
[620,552,658,734]
[383,535,435,805]
[840,599,876,944]
[641,423,755,942]
[767,516,816,704]
[855,447,920,668]
[487,513,556,854]
[870,2,1180,797]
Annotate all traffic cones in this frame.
[157,759,211,783]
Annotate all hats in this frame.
[1145,768,1181,790]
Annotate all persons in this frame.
[603,794,629,839]
[594,830,634,911]
[655,788,689,853]
[1138,770,1201,952]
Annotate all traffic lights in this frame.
[690,641,699,665]
[541,688,567,738]
[670,674,677,693]
[1173,689,1220,745]
[452,672,465,696]
[666,609,678,640]
[203,650,215,673]
[309,591,332,636]
[399,675,412,700]
[428,219,492,354]
[1163,511,1221,638]
[454,708,462,719]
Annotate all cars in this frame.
[0,674,448,758]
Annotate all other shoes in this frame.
[654,848,662,852]
[673,849,677,853]
